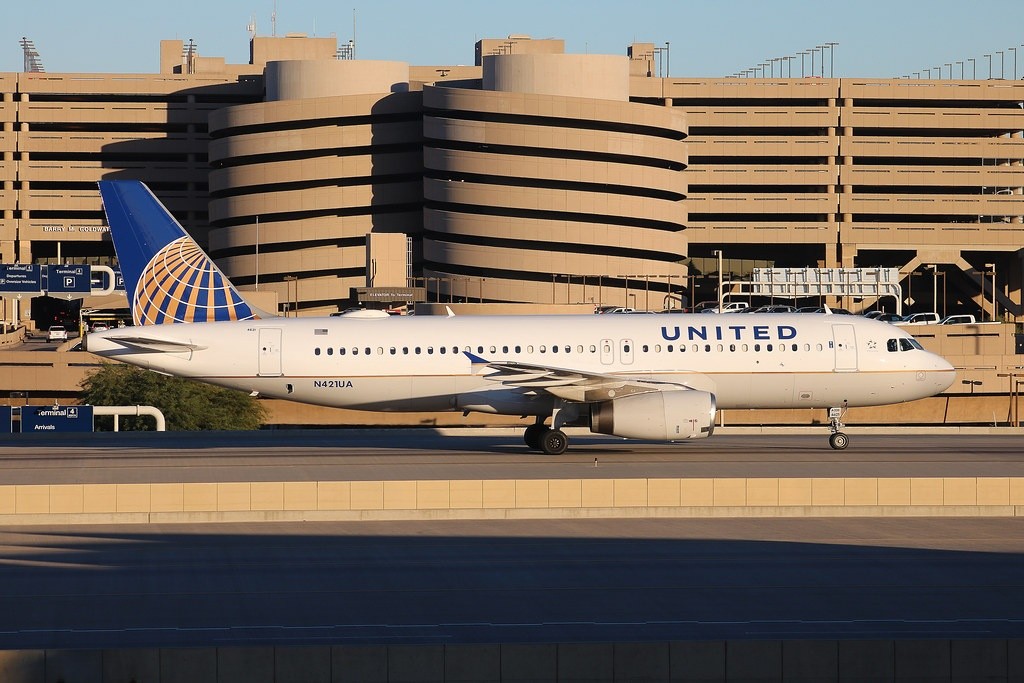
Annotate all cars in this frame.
[46,326,68,343]
[90,323,110,333]
[889,313,940,325]
[863,311,903,322]
[594,306,683,313]
[734,305,853,314]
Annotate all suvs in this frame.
[701,302,749,313]
[683,301,719,313]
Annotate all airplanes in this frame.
[82,181,958,457]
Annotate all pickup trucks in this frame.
[936,315,1001,325]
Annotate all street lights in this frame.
[714,250,723,313]
[928,265,937,313]
[985,263,996,322]
